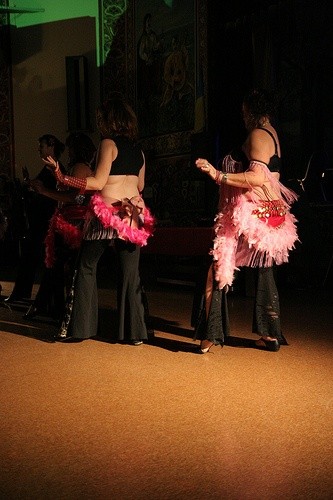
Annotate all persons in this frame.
[1,133,99,322]
[41,96,154,346]
[188,87,301,355]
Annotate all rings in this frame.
[206,164,209,167]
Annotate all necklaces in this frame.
[259,121,271,126]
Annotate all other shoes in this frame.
[195,340,224,354]
[249,337,280,351]
[4,293,25,303]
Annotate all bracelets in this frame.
[215,170,227,187]
